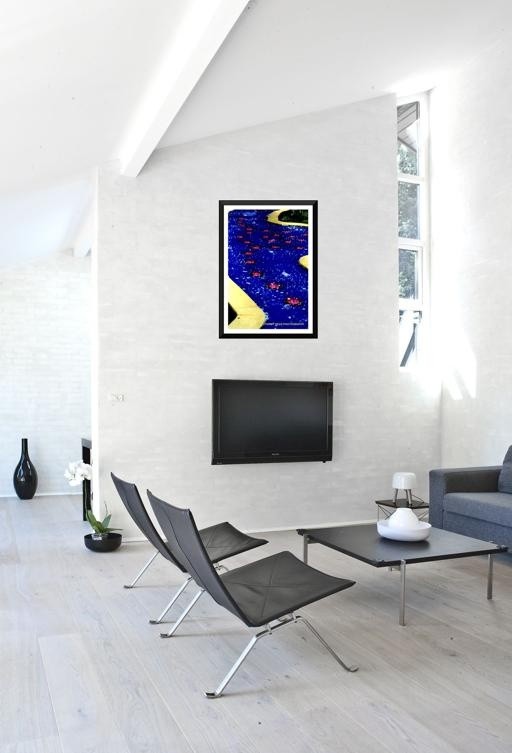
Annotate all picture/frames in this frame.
[219,199,318,338]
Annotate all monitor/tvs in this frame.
[212,379,333,465]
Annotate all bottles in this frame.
[388,508,419,528]
[13,437,39,500]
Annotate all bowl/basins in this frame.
[377,519,431,542]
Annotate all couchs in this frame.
[429,466,512,553]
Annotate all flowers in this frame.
[64,460,124,534]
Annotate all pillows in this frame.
[497,445,512,492]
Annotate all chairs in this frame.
[147,490,356,697]
[112,473,269,624]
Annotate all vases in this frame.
[84,533,121,552]
[13,438,38,500]
